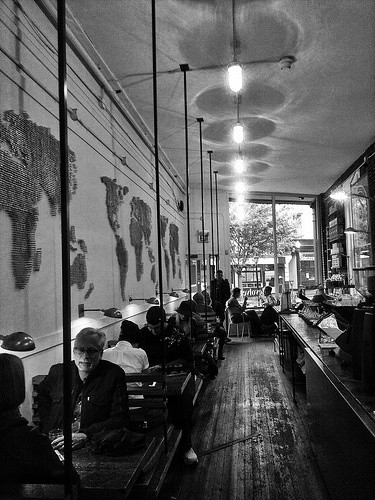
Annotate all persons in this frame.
[259,286,278,327]
[227,288,262,335]
[210,270,231,312]
[37,327,129,453]
[98,305,198,465]
[0,353,81,500]
[167,289,232,380]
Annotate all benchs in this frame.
[32,312,216,455]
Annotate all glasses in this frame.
[147,327,160,333]
[73,346,102,356]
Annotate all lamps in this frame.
[227,41,243,93]
[172,288,190,293]
[78,304,122,319]
[0,332,36,351]
[330,189,369,201]
[129,297,160,305]
[233,145,244,174]
[343,227,369,235]
[156,290,179,298]
[232,94,244,144]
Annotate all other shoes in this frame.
[184,448,198,462]
[226,339,231,342]
[219,356,224,360]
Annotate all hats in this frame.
[174,301,190,315]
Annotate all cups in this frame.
[48,428,63,454]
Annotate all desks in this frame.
[47,429,156,497]
[143,371,191,395]
[194,342,206,356]
[208,326,216,334]
[245,306,265,309]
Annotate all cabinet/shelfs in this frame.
[325,208,348,288]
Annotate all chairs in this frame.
[226,306,251,340]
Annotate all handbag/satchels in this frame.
[89,429,146,455]
